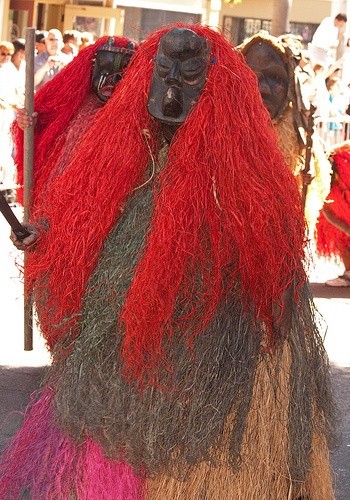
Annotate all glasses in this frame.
[0,53,12,56]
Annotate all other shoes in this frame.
[325,270,350,287]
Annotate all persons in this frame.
[0,29,94,207]
[0,21,342,494]
[326,247,350,287]
[10,35,139,208]
[295,14,349,146]
[231,29,331,263]
